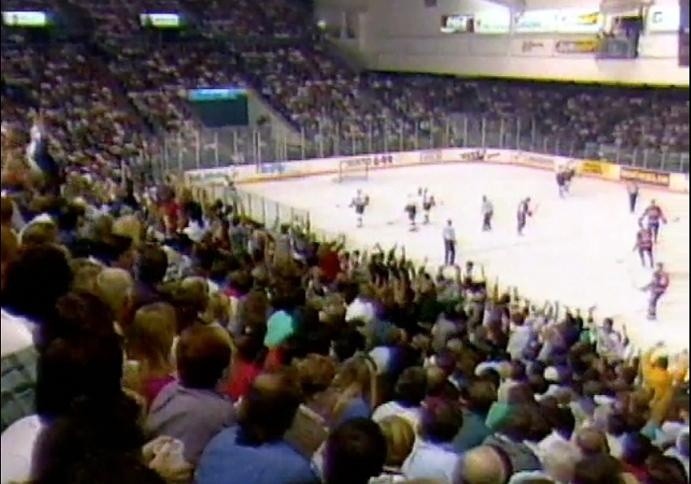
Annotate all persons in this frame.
[516,197,533,235]
[350,188,368,225]
[481,195,493,229]
[404,193,416,230]
[640,261,669,319]
[346,241,436,319]
[626,178,637,210]
[419,188,436,223]
[633,217,654,269]
[437,263,630,358]
[556,169,575,195]
[644,199,667,242]
[443,219,456,265]
